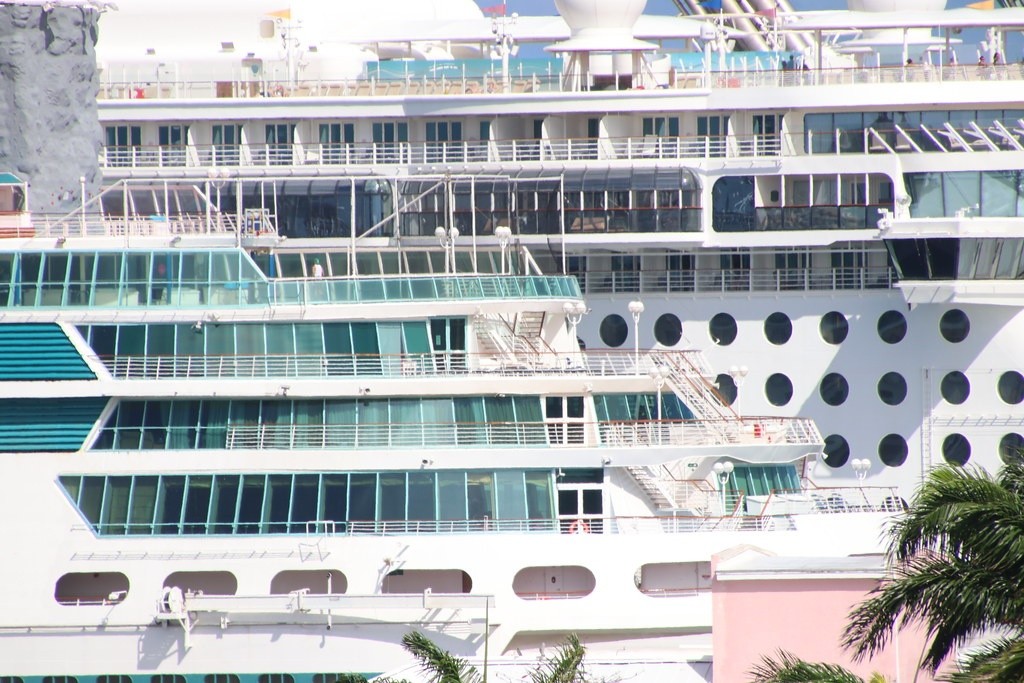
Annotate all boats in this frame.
[0,172,1024,683]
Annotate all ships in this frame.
[96,0,1024,516]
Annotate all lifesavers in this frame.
[569,520,589,536]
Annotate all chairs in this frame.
[403,82,420,95]
[511,80,526,92]
[327,83,345,96]
[403,360,417,377]
[527,80,542,90]
[418,83,434,95]
[895,132,912,153]
[996,134,1021,151]
[434,80,452,95]
[304,144,320,164]
[948,136,963,152]
[968,140,989,151]
[870,134,886,153]
[374,83,389,96]
[342,83,359,96]
[466,81,479,94]
[293,84,313,96]
[314,83,329,95]
[387,82,405,95]
[357,84,372,96]
[449,81,463,94]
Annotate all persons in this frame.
[992,52,1005,80]
[779,55,810,86]
[978,55,988,81]
[312,264,324,280]
[919,57,923,63]
[906,58,915,81]
[923,61,930,80]
